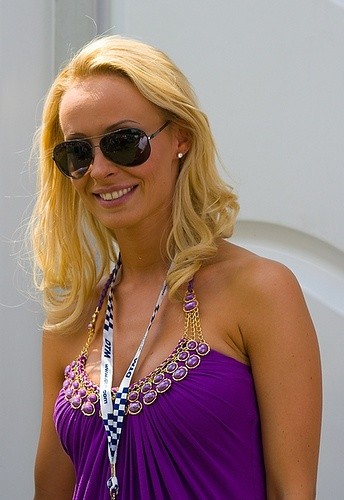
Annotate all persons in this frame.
[32,34,323,500]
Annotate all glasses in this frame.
[52,120,171,179]
